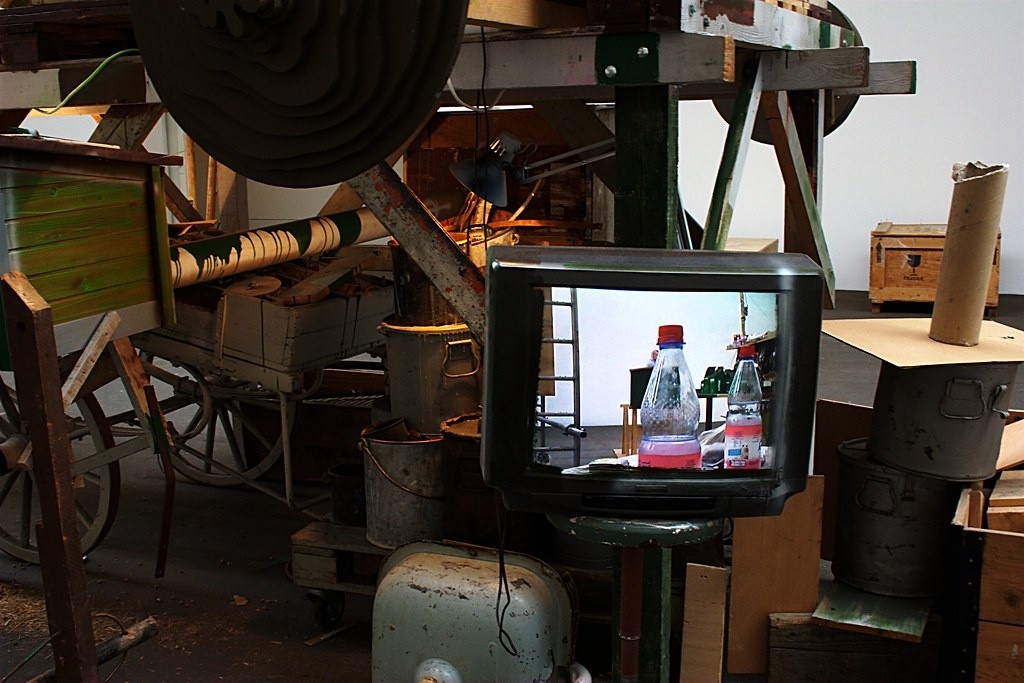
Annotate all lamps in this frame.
[449,130,615,208]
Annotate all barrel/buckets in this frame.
[831,437,964,597]
[440,411,504,539]
[386,232,473,326]
[865,361,1018,482]
[376,312,483,435]
[360,420,448,549]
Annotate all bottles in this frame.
[724,346,763,469]
[638,325,702,469]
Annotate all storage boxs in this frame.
[869,221,1000,315]
[0,133,183,371]
[128,224,397,393]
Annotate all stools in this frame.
[543,506,725,683]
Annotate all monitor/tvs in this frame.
[481,245,825,522]
